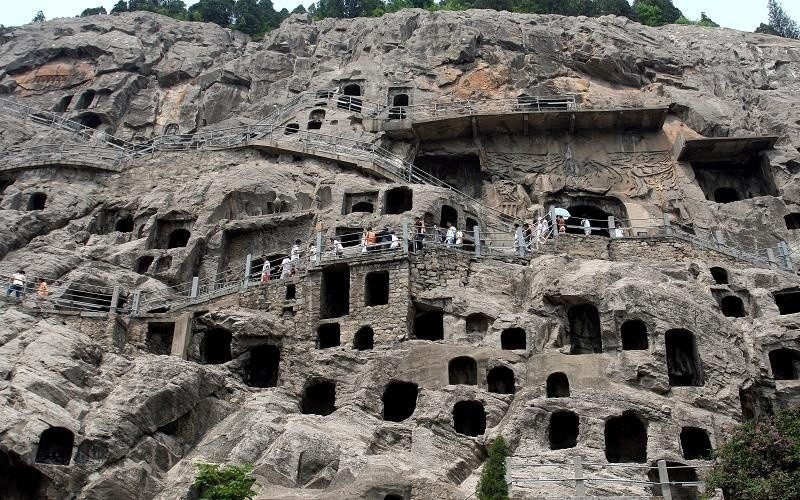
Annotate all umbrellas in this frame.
[548,207,571,220]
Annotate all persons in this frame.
[513,217,546,256]
[615,224,624,238]
[361,216,463,253]
[291,239,304,276]
[307,243,316,262]
[7,270,26,298]
[581,215,591,236]
[556,215,566,234]
[261,255,270,282]
[37,277,48,310]
[330,238,344,258]
[278,255,293,279]
